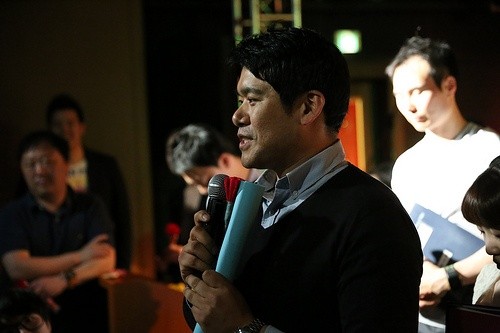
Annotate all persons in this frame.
[0,131,118,297]
[461,155,500,313]
[46,95,132,274]
[383,37,500,333]
[164,124,266,193]
[178,26,423,333]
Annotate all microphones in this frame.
[191,174,230,279]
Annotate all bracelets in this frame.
[239,317,262,332]
[445,264,461,289]
[62,268,75,283]
